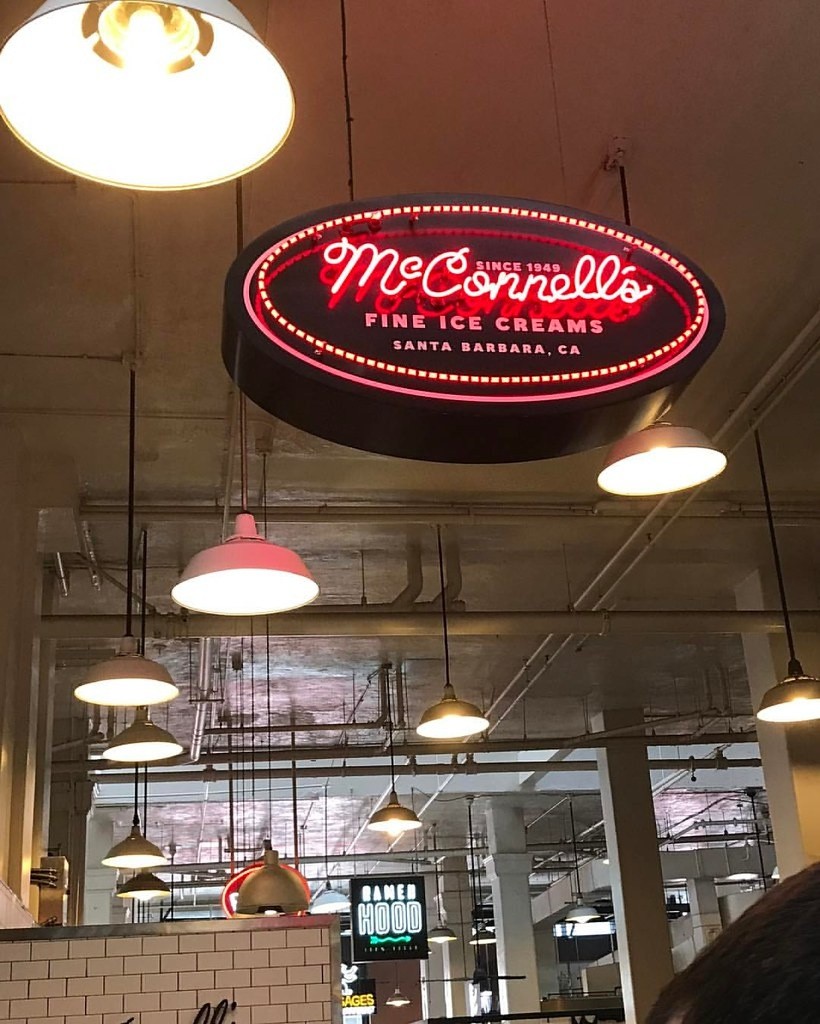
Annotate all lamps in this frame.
[753,427,820,722]
[234,613,310,914]
[565,792,600,923]
[70,365,180,707]
[414,524,491,738]
[171,178,323,617]
[385,962,411,1007]
[593,135,728,498]
[117,762,172,900]
[0,0,295,193]
[101,530,187,762]
[308,784,352,914]
[101,760,168,869]
[426,822,457,943]
[366,662,423,831]
[467,795,497,945]
